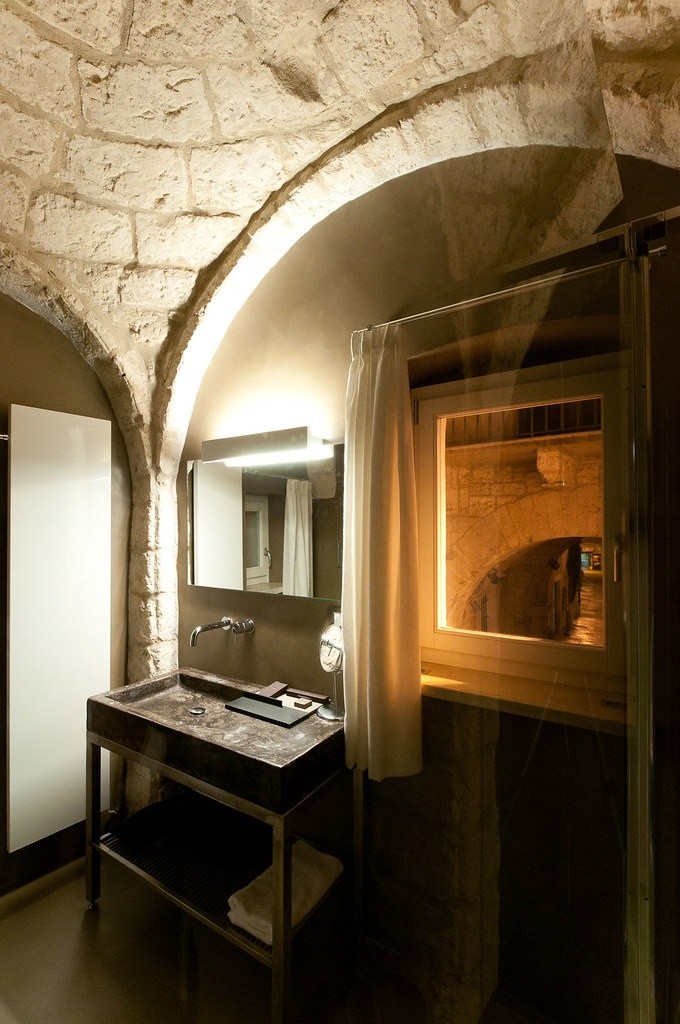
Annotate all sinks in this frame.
[85,666,346,814]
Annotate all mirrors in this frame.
[187,443,345,600]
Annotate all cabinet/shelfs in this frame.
[84,730,363,1024]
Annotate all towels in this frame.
[226,841,344,945]
[228,838,346,946]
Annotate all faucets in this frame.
[189,615,234,647]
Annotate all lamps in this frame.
[200,425,316,464]
[225,439,334,467]
[547,558,560,570]
[487,567,508,585]
